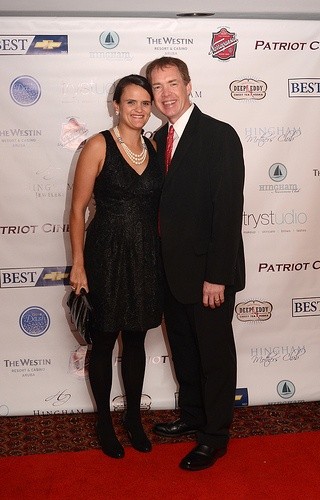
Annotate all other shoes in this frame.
[123,414,152,452]
[96,424,124,458]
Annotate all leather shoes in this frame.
[154,417,201,437]
[179,443,227,471]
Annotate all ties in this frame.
[165,125,175,172]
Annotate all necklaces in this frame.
[113,126,147,164]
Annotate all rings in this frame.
[215,299,220,303]
[72,286,76,290]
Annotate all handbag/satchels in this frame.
[67,288,98,345]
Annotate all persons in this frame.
[92,57,246,471]
[68,75,164,459]
